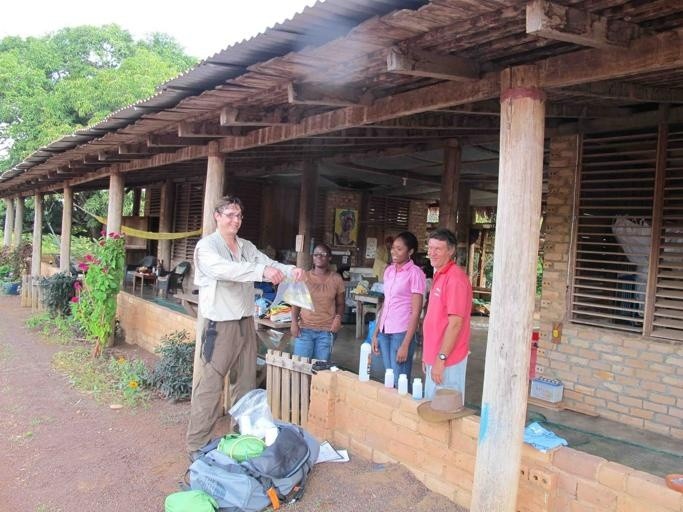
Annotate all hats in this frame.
[416,389,477,423]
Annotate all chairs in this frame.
[157,261,191,299]
[127,256,155,275]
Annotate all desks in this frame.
[353,292,387,340]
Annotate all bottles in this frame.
[411,378,424,399]
[358,342,373,384]
[397,372,408,397]
[383,367,395,389]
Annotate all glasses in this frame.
[222,212,243,220]
[313,252,329,257]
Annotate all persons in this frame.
[372,228,472,405]
[334,211,356,245]
[187,196,307,462]
[291,244,345,361]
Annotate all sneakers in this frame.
[188,451,203,463]
